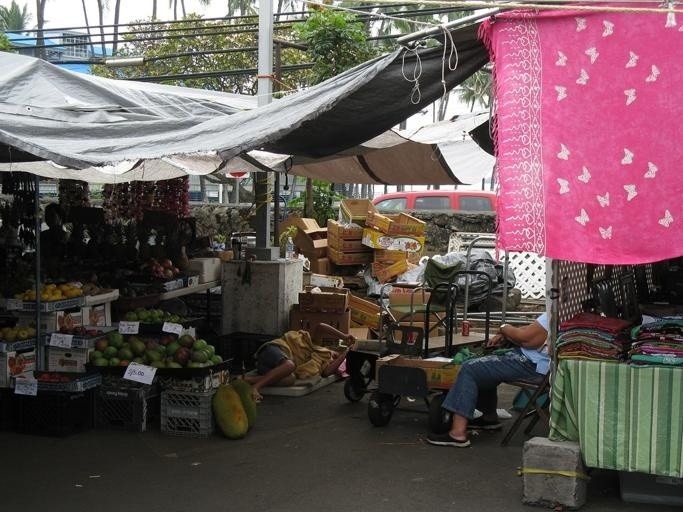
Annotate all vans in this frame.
[373,191,499,214]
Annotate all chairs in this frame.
[496,369,550,447]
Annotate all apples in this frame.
[122,305,181,323]
[13,283,84,302]
[0,327,36,343]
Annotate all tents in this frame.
[2,113,502,186]
[1,21,501,166]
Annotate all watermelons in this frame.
[92,333,223,368]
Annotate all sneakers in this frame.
[467,417,503,430]
[426,432,471,448]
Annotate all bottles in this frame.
[286,236,293,260]
[462,321,469,336]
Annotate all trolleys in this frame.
[367,269,498,432]
[344,282,459,404]
[379,236,510,346]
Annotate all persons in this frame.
[237,323,357,402]
[425,312,551,449]
[38,202,72,270]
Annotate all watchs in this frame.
[499,324,509,332]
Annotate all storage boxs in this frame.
[188,256,221,283]
[362,229,425,251]
[371,265,389,277]
[39,370,111,392]
[85,288,119,306]
[0,350,37,389]
[93,381,159,435]
[295,225,328,257]
[325,246,370,267]
[326,219,362,240]
[371,248,410,263]
[14,311,54,334]
[311,259,331,274]
[82,305,112,328]
[387,310,438,340]
[341,198,378,225]
[161,390,215,439]
[22,295,84,311]
[364,211,425,236]
[55,312,83,333]
[45,347,86,373]
[289,304,351,338]
[2,338,37,353]
[387,292,447,322]
[23,390,90,439]
[327,234,370,254]
[375,353,465,390]
[1,390,21,430]
[308,272,363,290]
[387,283,426,293]
[153,357,234,391]
[279,213,320,231]
[298,285,350,314]
[378,259,409,283]
[349,295,385,332]
[349,326,371,342]
